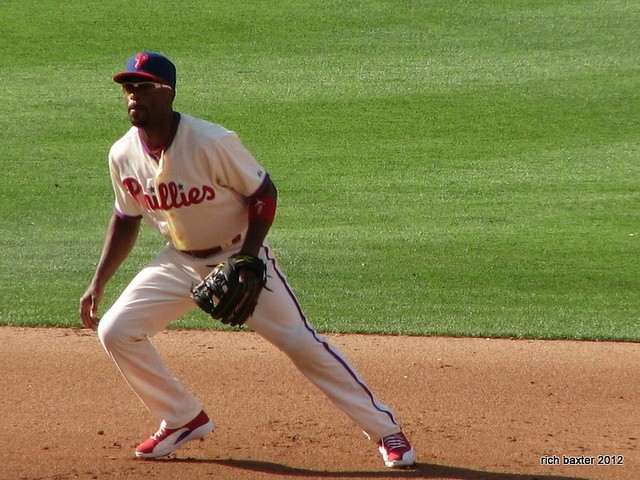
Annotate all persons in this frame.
[80,52,415,469]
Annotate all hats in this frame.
[113,52,176,89]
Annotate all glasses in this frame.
[122,82,173,92]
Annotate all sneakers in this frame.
[377,432,414,467]
[135,410,214,457]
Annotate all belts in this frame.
[180,234,241,258]
